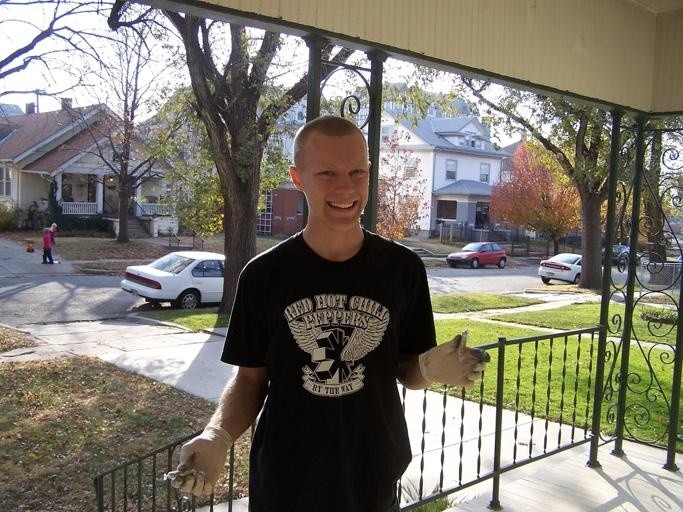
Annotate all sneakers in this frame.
[41,260,55,265]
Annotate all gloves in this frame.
[417,330,492,390]
[167,421,235,499]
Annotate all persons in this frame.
[164,114,493,512]
[41,228,54,265]
[48,222,57,246]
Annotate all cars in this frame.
[599,244,641,270]
[537,252,603,289]
[443,240,507,271]
[119,249,227,312]
[668,255,682,274]
